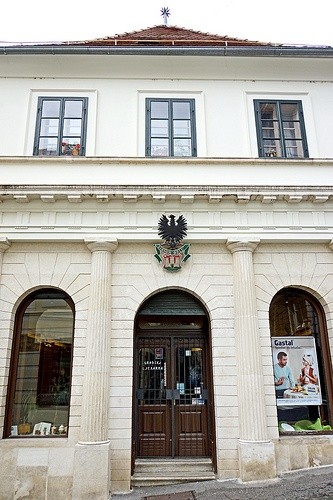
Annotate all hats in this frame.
[303,354,314,366]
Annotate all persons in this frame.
[299,354,317,387]
[273,351,296,397]
